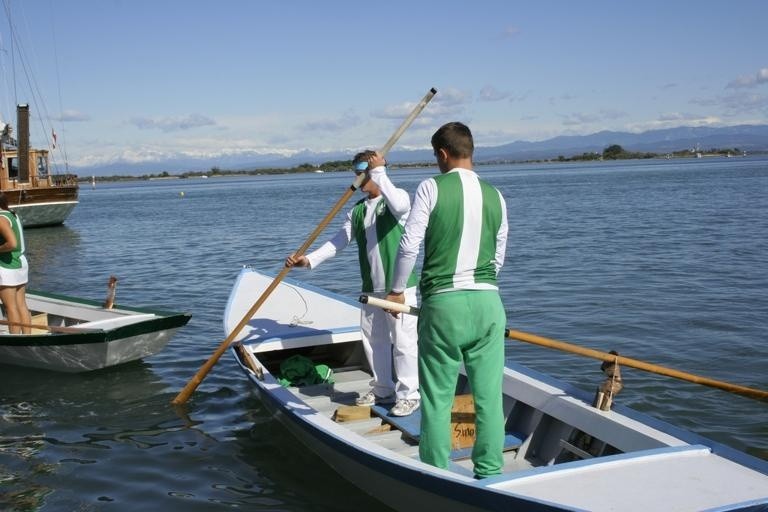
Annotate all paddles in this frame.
[173,88,438,405]
[1,320,106,335]
[359,294,767,401]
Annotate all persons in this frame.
[383,122,509,480]
[284,150,422,417]
[0,190,32,335]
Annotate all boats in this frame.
[223,263,768,512]
[0,288,193,375]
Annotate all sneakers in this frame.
[355,390,395,406]
[390,399,421,417]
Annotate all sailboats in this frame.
[0,0,80,229]
